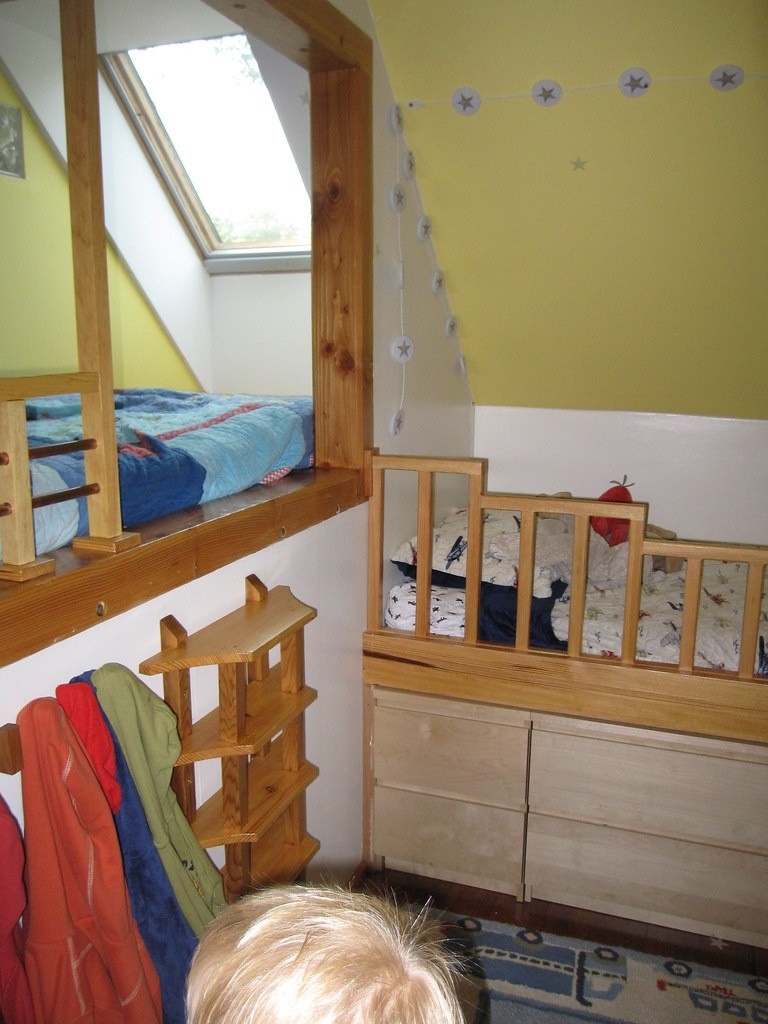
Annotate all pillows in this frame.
[391,510,612,601]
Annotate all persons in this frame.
[183,884,466,1024]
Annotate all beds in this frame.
[355,455,767,978]
[2,3,376,918]
